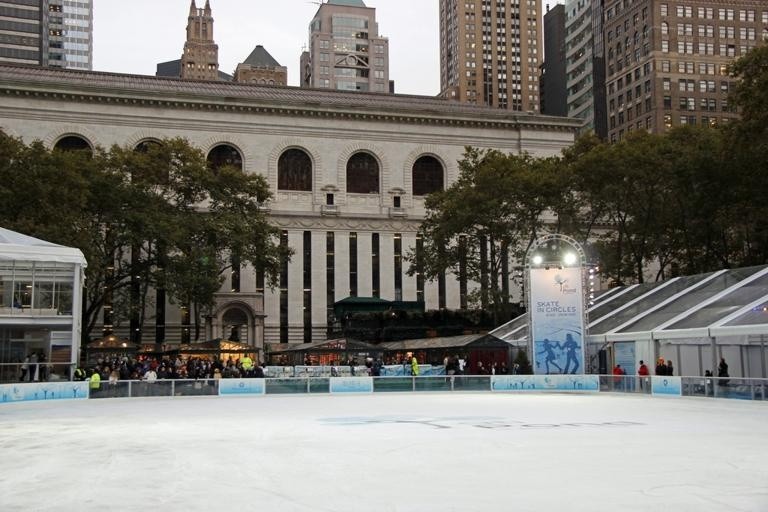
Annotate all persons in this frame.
[535,338,563,374]
[16,351,730,397]
[556,332,581,374]
